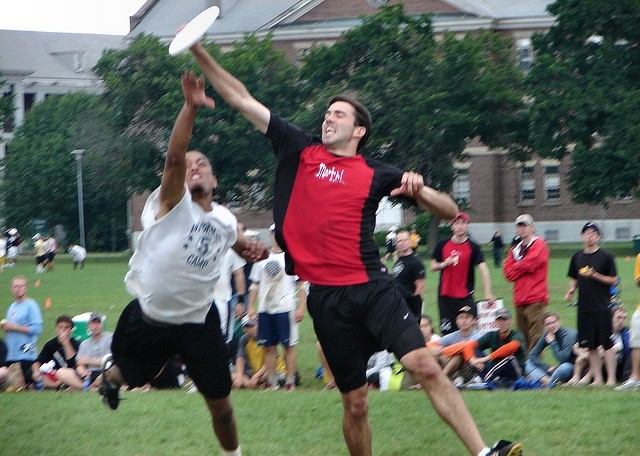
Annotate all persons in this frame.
[8,228,20,267]
[66,242,87,272]
[524,312,577,388]
[567,306,627,385]
[463,308,525,390]
[230,313,288,389]
[615,252,640,390]
[42,233,56,272]
[487,230,506,268]
[408,305,488,391]
[0,236,8,271]
[32,316,80,389]
[430,212,495,335]
[399,315,441,390]
[316,340,396,392]
[411,228,419,253]
[503,215,549,364]
[0,337,10,388]
[58,313,117,390]
[174,23,524,455]
[214,245,247,345]
[0,275,47,392]
[381,225,400,265]
[247,223,310,394]
[98,67,268,455]
[31,233,44,273]
[391,231,426,324]
[564,223,622,388]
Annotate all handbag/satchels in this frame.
[388,362,416,390]
[477,297,506,334]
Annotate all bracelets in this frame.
[236,293,245,305]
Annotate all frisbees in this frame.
[169,5,220,58]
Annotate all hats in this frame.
[457,306,476,317]
[515,214,535,226]
[241,313,258,326]
[451,212,471,224]
[90,312,102,322]
[494,307,511,320]
[582,222,601,234]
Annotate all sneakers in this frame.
[260,383,280,392]
[99,353,120,409]
[613,379,640,390]
[283,383,294,391]
[486,439,522,456]
